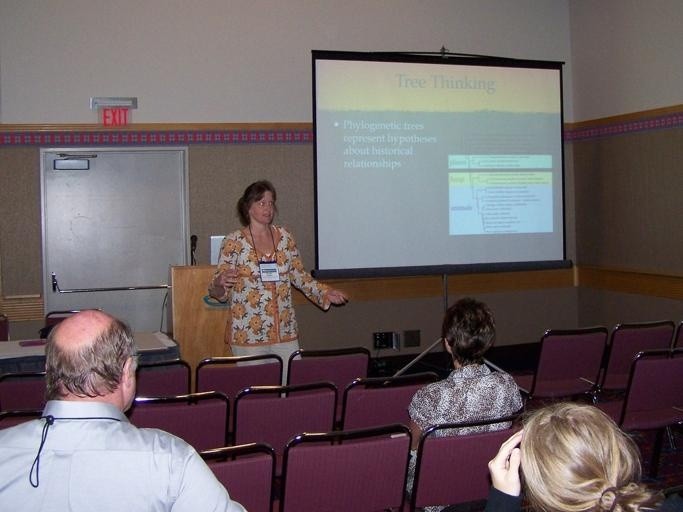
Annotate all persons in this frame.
[209,177,350,397]
[482,399,670,511]
[0,308,249,511]
[395,296,517,511]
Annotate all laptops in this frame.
[210,236,226,265]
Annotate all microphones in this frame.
[190,235,197,250]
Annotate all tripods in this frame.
[382,272,510,385]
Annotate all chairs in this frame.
[0,313,682,512]
